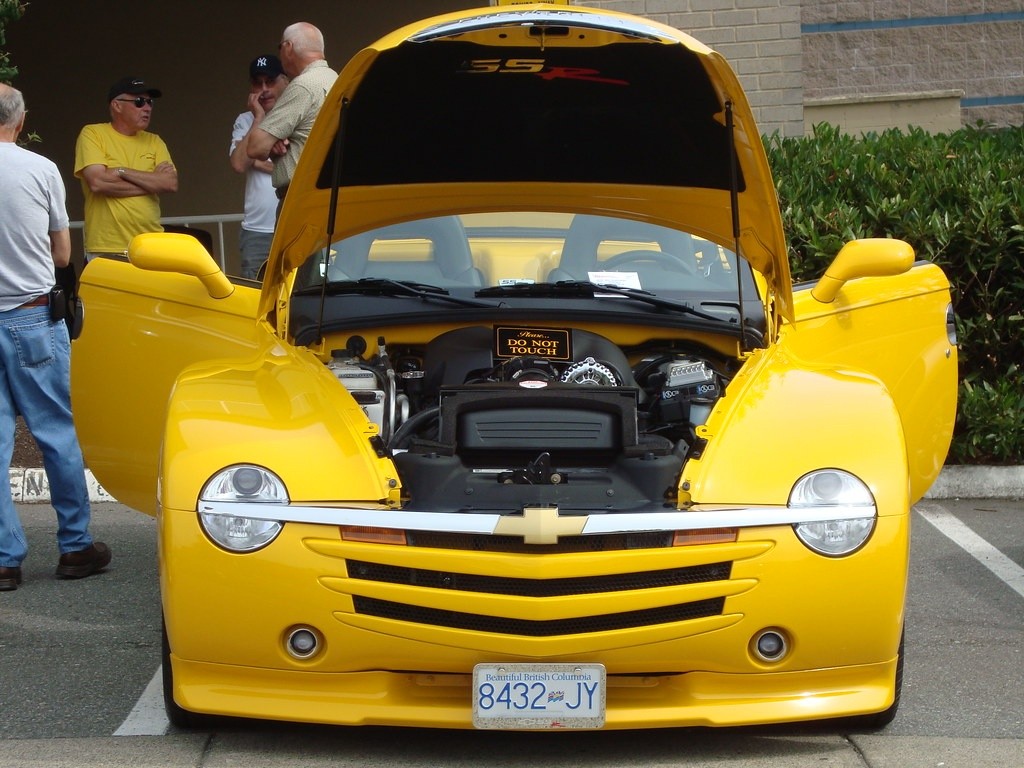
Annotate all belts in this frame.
[22,294,49,305]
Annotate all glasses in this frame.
[115,97,154,108]
[278,40,293,49]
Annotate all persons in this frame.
[229,55,290,282]
[246,22,339,234]
[0,82,112,590]
[74,76,179,262]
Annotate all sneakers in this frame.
[0,567,21,590]
[57,542,112,577]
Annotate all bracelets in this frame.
[118,167,124,176]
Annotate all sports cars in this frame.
[68,1,959,734]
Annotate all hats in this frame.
[109,76,163,101]
[250,55,282,79]
